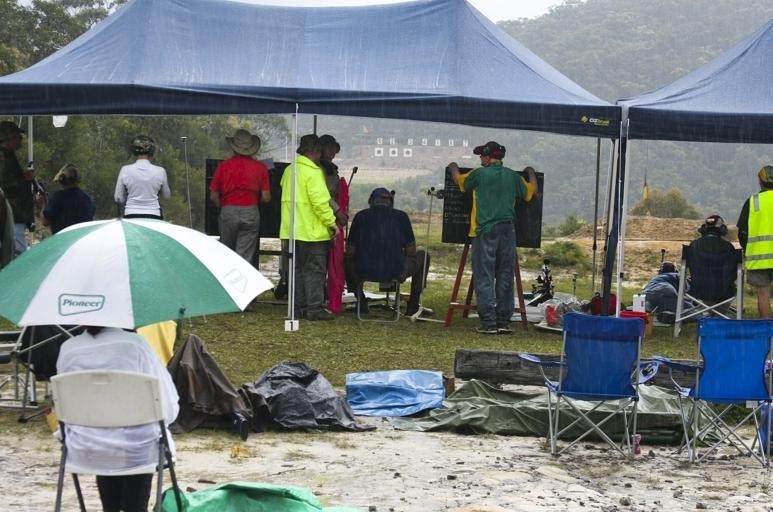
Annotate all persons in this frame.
[53,326,180,512]
[210,129,272,311]
[1,122,96,267]
[280,135,339,321]
[448,141,538,333]
[644,262,692,324]
[346,188,433,318]
[686,214,738,316]
[114,135,170,220]
[737,166,773,320]
[316,135,348,232]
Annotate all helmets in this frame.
[704,215,724,228]
[53,164,81,185]
[131,135,155,155]
[660,262,676,273]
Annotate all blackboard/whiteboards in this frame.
[204,159,292,238]
[441,166,544,248]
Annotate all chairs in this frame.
[517,312,645,463]
[653,315,773,468]
[0,332,39,407]
[50,369,183,512]
[673,259,742,339]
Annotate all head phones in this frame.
[698,216,728,237]
[130,139,155,155]
[58,164,80,183]
[368,191,394,205]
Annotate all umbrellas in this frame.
[0,218,275,332]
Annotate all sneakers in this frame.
[346,301,370,312]
[475,326,499,334]
[307,309,335,320]
[498,323,513,333]
[407,301,434,316]
[295,307,308,318]
[657,314,676,323]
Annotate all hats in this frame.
[0,121,27,141]
[369,188,393,204]
[297,134,321,152]
[225,130,261,156]
[758,166,773,184]
[319,135,341,154]
[474,141,505,159]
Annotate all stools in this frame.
[355,276,401,323]
